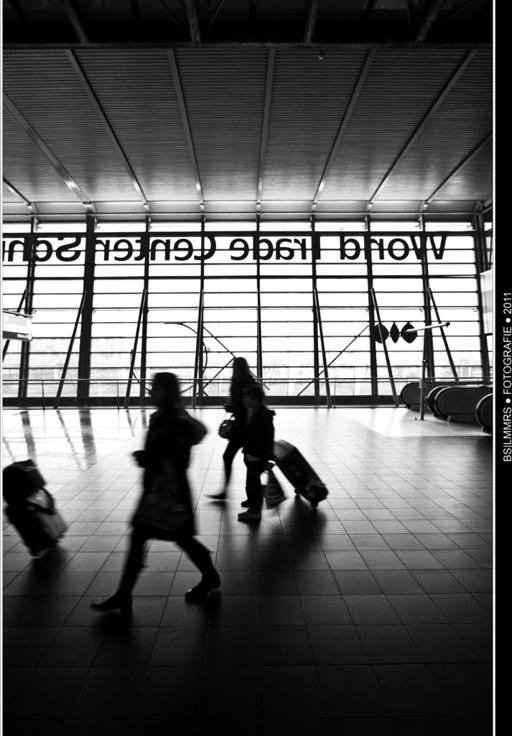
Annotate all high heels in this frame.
[89,593,134,618]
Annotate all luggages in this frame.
[270,439,328,509]
[5,487,69,559]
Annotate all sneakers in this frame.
[207,492,263,523]
[185,572,222,601]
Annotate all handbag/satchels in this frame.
[219,414,240,438]
[3,459,47,503]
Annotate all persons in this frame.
[202,357,264,501]
[88,371,221,613]
[236,385,277,524]
